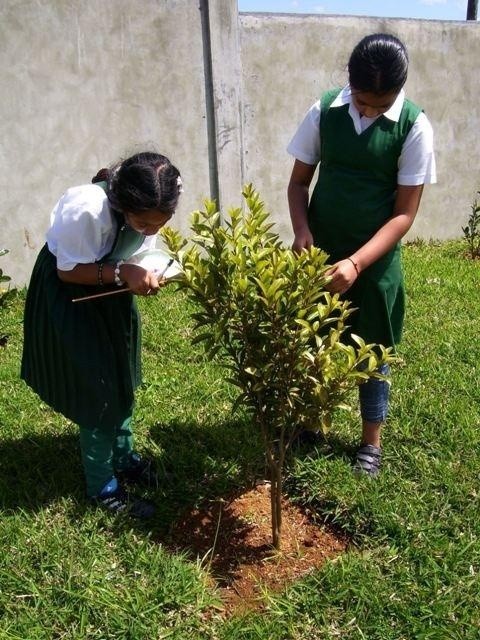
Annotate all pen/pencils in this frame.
[147,259,174,295]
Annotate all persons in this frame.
[286,31,438,484]
[19,148,184,524]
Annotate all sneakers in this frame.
[87,478,159,520]
[354,442,383,474]
[113,452,175,487]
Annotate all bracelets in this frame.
[98,263,107,292]
[346,256,360,279]
[113,260,126,287]
